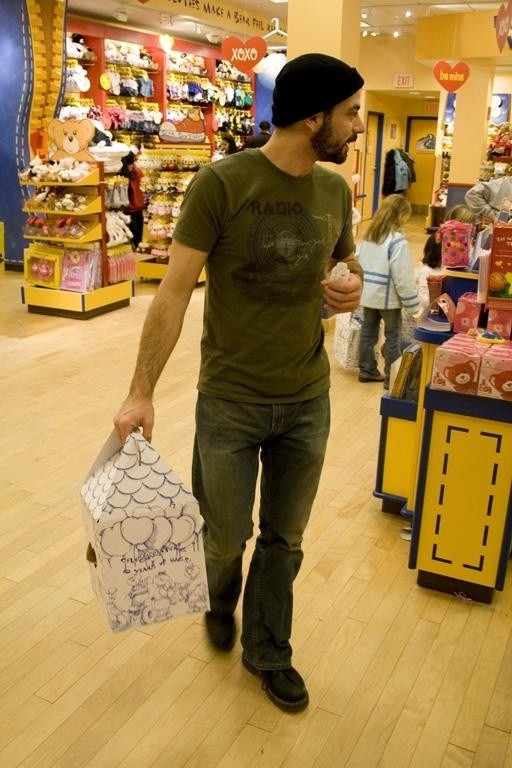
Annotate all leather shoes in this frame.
[204,608,237,653]
[359,372,385,382]
[243,656,309,713]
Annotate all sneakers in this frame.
[159,108,205,142]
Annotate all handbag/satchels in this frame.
[334,310,363,369]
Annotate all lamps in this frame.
[207,26,219,44]
[112,3,128,22]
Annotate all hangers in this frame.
[261,17,288,50]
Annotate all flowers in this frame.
[488,123,512,142]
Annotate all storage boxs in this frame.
[486,300,512,339]
[441,224,473,266]
[488,225,512,298]
[453,292,481,333]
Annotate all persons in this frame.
[123,162,145,248]
[465,176,512,224]
[355,194,420,383]
[113,55,365,712]
[380,234,444,358]
[211,126,229,162]
[116,152,137,205]
[222,135,240,157]
[240,121,272,152]
[444,207,476,224]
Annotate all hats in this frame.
[272,54,364,123]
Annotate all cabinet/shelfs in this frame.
[19,161,132,321]
[135,141,215,282]
[373,269,512,605]
[54,12,255,151]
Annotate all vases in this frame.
[493,155,512,163]
